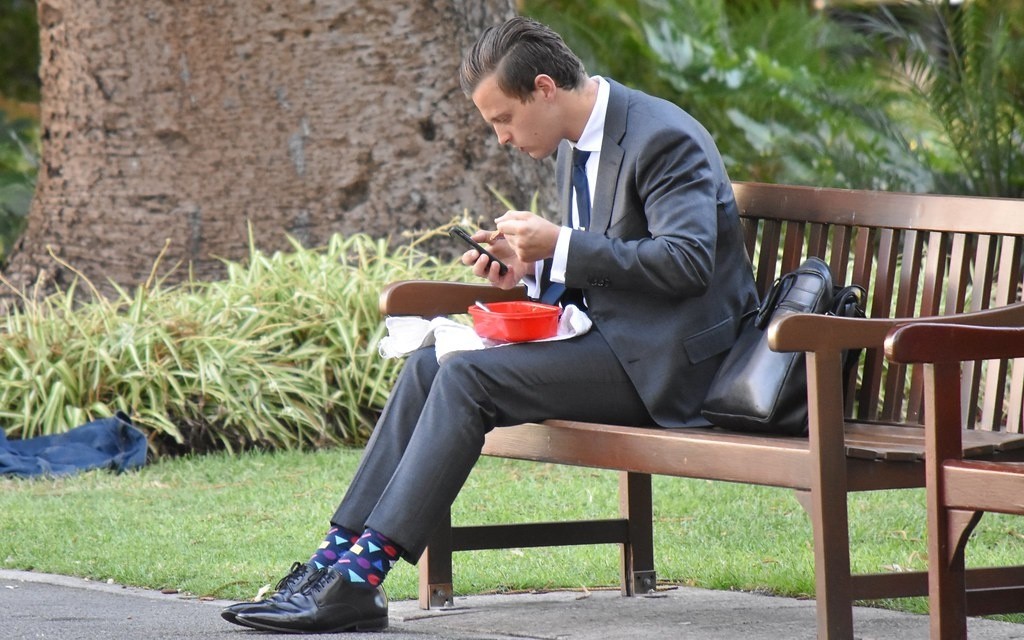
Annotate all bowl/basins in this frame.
[467,300,563,341]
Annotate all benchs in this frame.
[376,178,1024,639]
[882,322,1024,639]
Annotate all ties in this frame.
[540,146,593,305]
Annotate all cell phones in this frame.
[449,227,507,276]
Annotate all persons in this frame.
[212,14,772,638]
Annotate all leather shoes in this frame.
[220,562,390,633]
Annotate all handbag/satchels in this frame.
[698,255,866,437]
